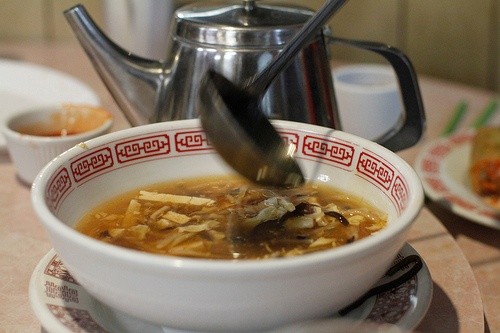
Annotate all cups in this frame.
[329,62,412,146]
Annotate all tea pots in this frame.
[63,0,426,172]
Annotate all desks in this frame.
[1,43,500,333]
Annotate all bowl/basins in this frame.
[3,99,114,187]
[30,119,426,331]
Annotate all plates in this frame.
[0,55,101,151]
[30,244,434,333]
[412,124,500,231]
